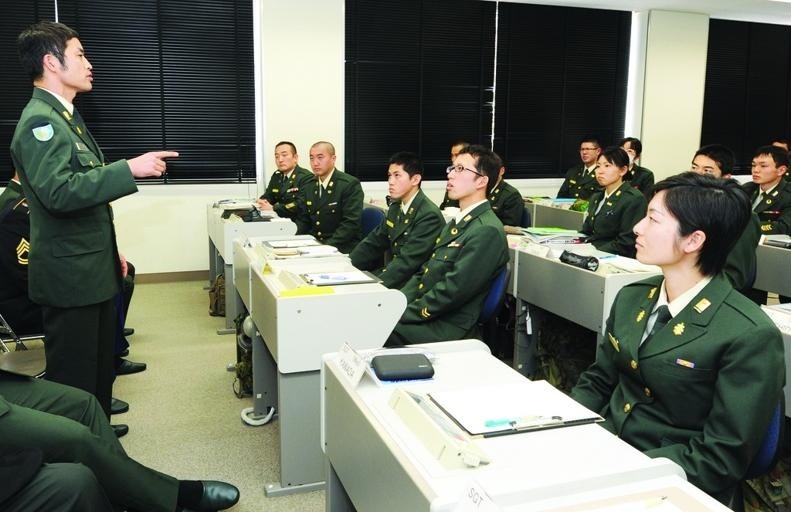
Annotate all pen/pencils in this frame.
[485,415,550,425]
[320,275,347,280]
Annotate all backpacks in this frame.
[209,276,225,317]
[232,313,252,398]
[743,458,791,512]
[533,317,587,392]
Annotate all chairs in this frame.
[2,333,46,344]
[0,313,47,379]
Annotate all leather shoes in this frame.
[107,328,147,438]
[183,480,240,510]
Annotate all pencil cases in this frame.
[560,251,599,272]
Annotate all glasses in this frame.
[446,164,483,177]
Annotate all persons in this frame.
[690,143,762,291]
[9,19,180,422]
[576,148,647,259]
[568,172,786,506]
[0,370,240,512]
[383,144,510,348]
[0,166,146,434]
[347,151,446,290]
[487,158,523,226]
[255,142,315,217]
[557,135,791,238]
[439,137,473,208]
[292,141,364,254]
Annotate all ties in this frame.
[583,169,589,178]
[321,184,326,198]
[400,210,404,223]
[283,175,289,192]
[639,304,672,352]
[449,218,457,231]
